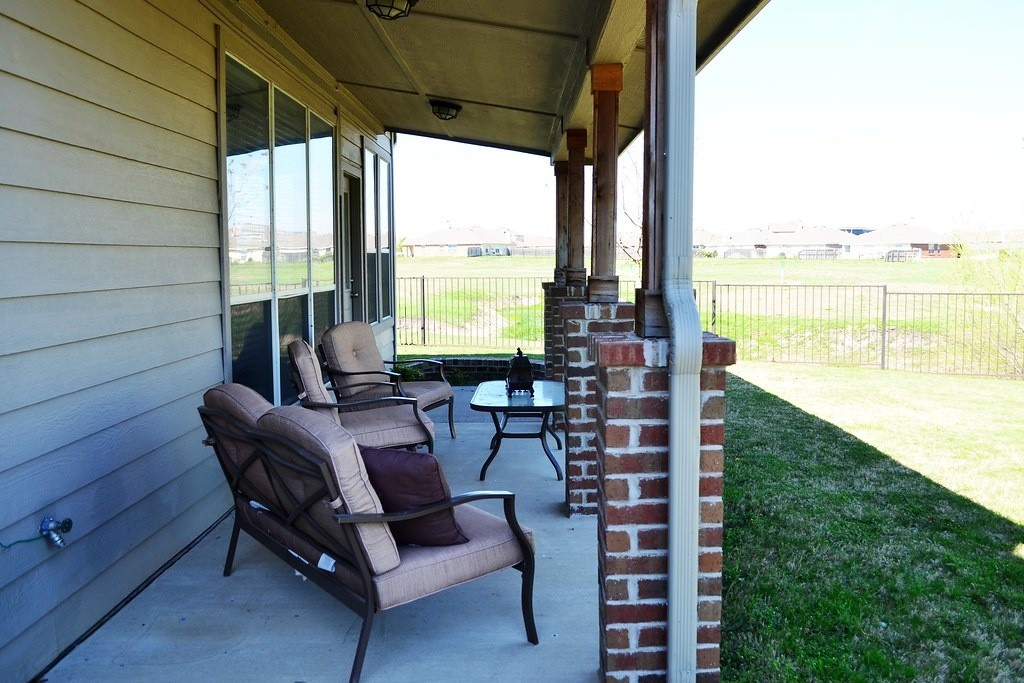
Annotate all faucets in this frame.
[41,517,72,550]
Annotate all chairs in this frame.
[287,339,435,455]
[323,320,455,440]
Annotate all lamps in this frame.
[430,99,464,120]
[365,0,419,21]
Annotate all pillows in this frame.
[358,444,468,545]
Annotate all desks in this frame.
[469,380,567,481]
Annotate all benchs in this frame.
[197,381,538,683]
